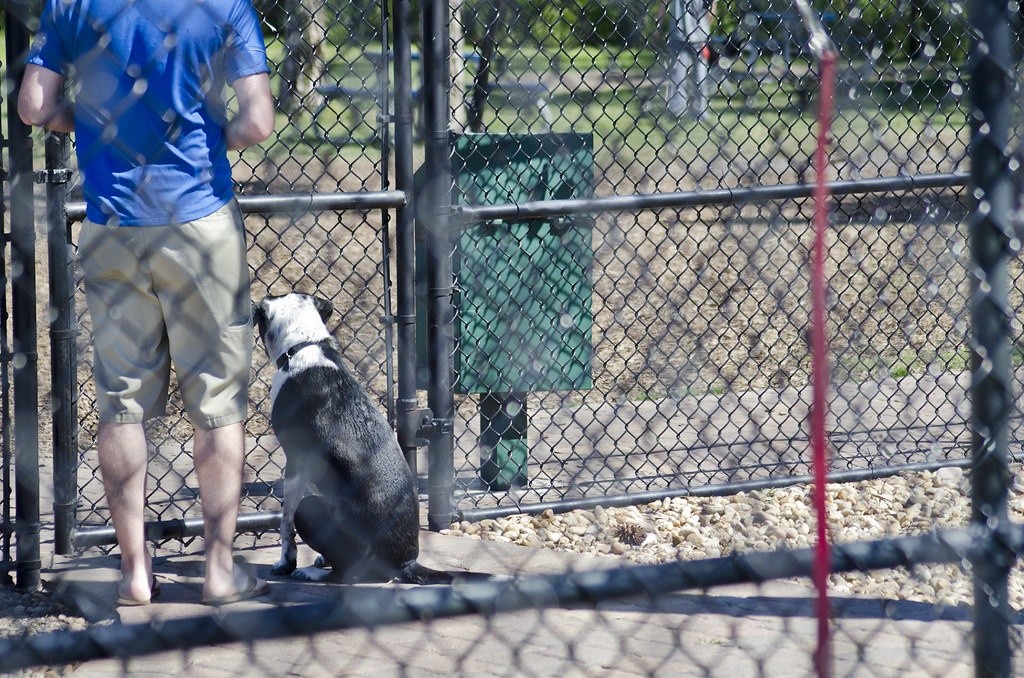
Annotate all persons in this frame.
[17,0,276,607]
[655,0,718,118]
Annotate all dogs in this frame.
[252,292,524,585]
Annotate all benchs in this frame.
[710,34,743,82]
[466,82,551,134]
[311,84,424,148]
[838,35,872,82]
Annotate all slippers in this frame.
[118,573,162,607]
[203,574,272,605]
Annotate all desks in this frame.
[362,50,482,141]
[741,10,836,81]
